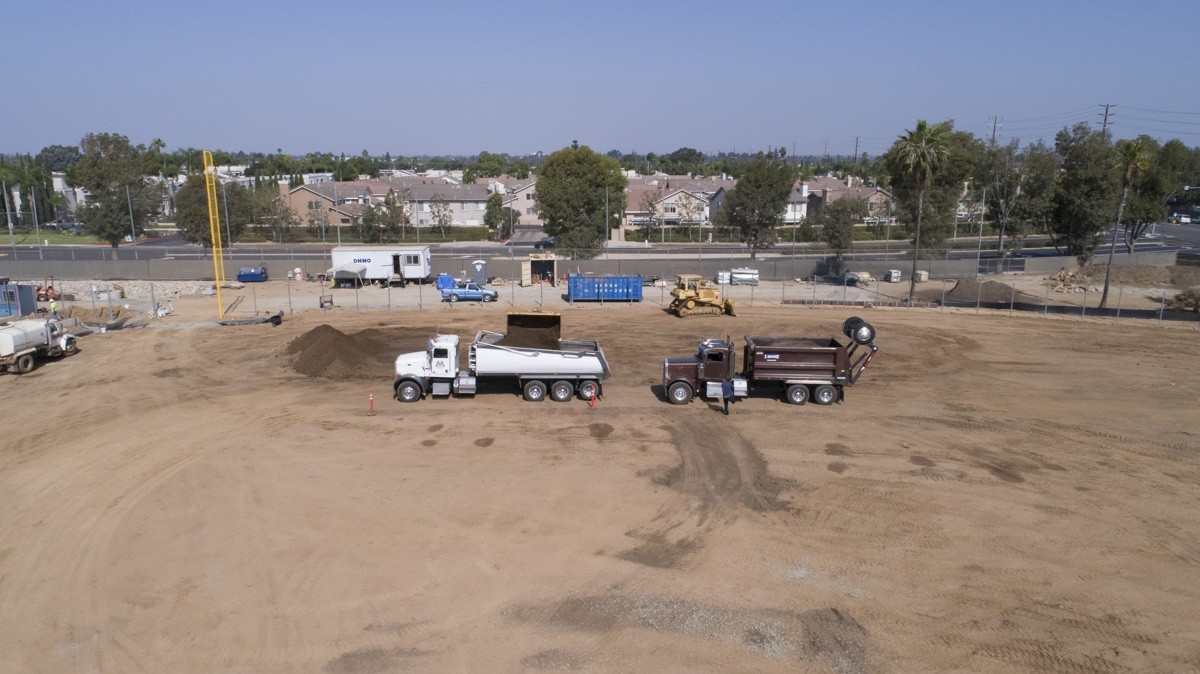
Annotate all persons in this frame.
[721,376,735,415]
[49,298,63,321]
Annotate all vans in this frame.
[1167,214,1191,224]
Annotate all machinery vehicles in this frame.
[34,276,61,302]
[669,274,736,317]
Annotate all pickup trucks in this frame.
[441,282,498,302]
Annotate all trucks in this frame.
[392,329,612,402]
[662,334,852,405]
[1,319,77,373]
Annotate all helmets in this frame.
[49,299,53,302]
